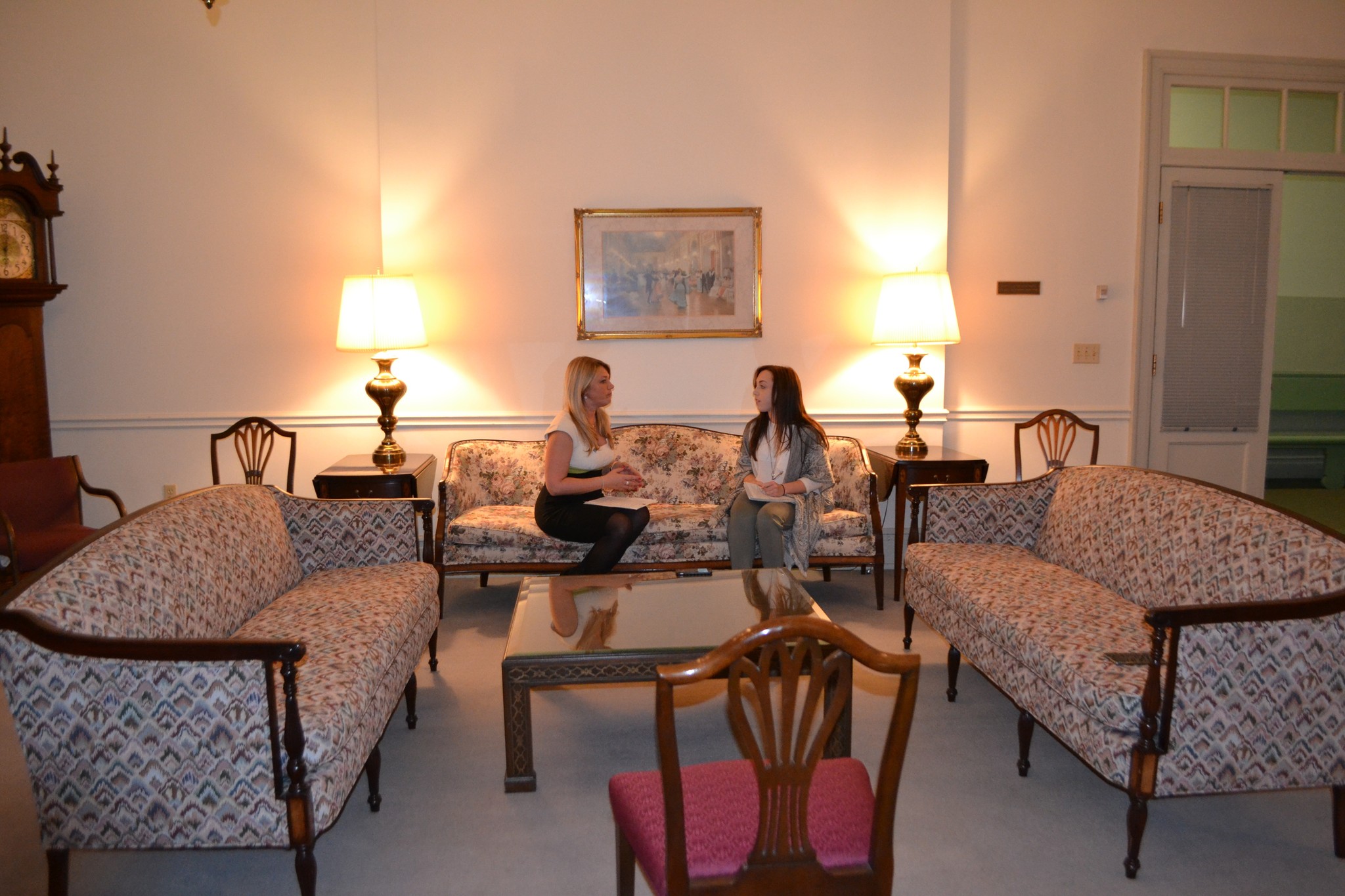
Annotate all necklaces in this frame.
[768,425,784,481]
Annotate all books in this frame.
[743,481,795,502]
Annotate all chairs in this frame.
[1014,409,1100,481]
[609,615,921,896]
[0,455,126,589]
[210,416,296,494]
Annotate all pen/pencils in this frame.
[761,471,784,492]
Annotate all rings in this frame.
[626,481,629,485]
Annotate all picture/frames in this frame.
[573,207,762,341]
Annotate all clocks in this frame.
[0,127,69,463]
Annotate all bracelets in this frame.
[611,461,619,470]
[780,484,785,496]
[601,476,604,490]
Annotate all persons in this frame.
[535,356,651,576]
[547,576,638,653]
[741,569,815,622]
[707,366,834,577]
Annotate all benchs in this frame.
[0,483,440,896]
[904,465,1345,879]
[435,424,884,618]
[1267,373,1345,489]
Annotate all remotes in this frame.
[676,568,712,576]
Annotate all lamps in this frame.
[336,269,429,467]
[872,267,961,451]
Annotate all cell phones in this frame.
[1102,649,1169,666]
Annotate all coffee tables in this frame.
[501,567,853,793]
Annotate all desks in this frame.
[865,446,989,602]
[313,453,437,498]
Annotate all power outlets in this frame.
[164,484,177,499]
